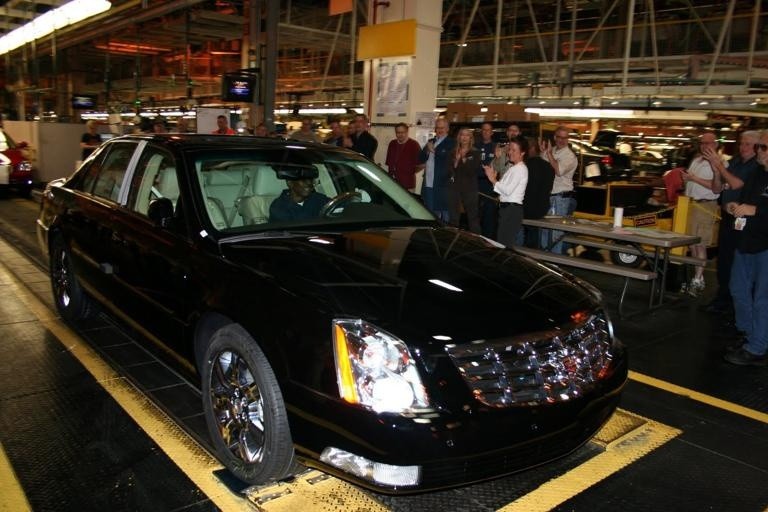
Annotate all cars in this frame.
[563,134,692,266]
[31,132,630,493]
[0,125,33,193]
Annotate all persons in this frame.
[109,105,122,134]
[665,121,768,367]
[80,119,103,161]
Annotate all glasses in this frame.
[752,143,768,154]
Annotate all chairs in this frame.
[151,167,225,232]
[235,163,289,225]
[198,167,253,226]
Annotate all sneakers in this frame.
[723,336,750,349]
[678,285,741,331]
[724,350,767,367]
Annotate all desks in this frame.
[520,215,703,312]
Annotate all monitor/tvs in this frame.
[72,94,96,109]
[222,76,256,103]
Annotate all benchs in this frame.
[557,233,714,309]
[508,246,659,320]
